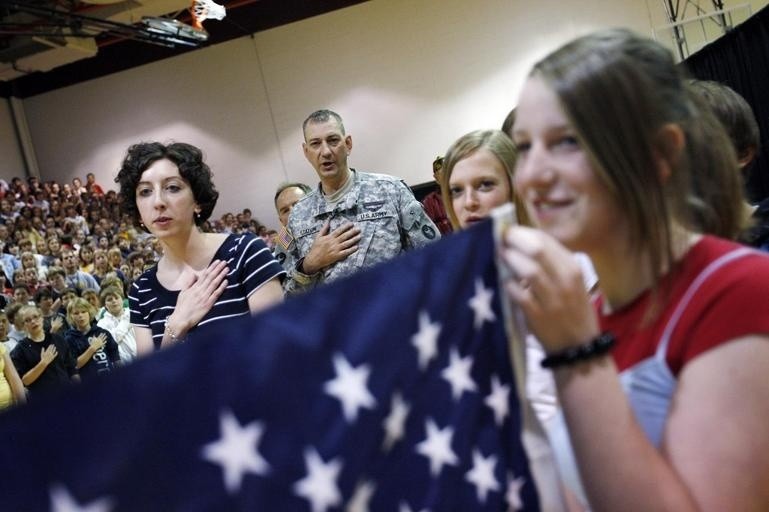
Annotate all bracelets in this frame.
[164,316,185,345]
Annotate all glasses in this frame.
[434,158,444,168]
[21,313,42,327]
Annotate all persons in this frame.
[425,128,516,238]
[113,142,285,359]
[201,207,279,249]
[274,110,443,300]
[503,26,768,512]
[1,172,164,407]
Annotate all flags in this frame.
[0,205,564,511]
